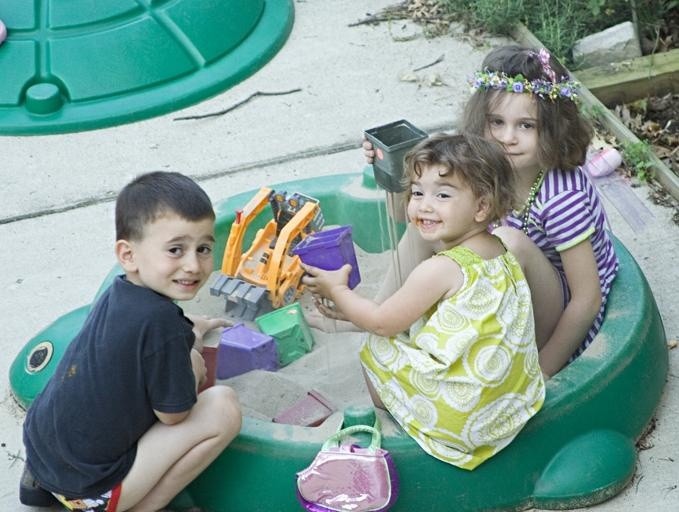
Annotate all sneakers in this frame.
[17,466,58,507]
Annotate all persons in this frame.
[300,132,546,471]
[361,45,620,377]
[22,169,245,512]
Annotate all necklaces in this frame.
[488,167,544,236]
